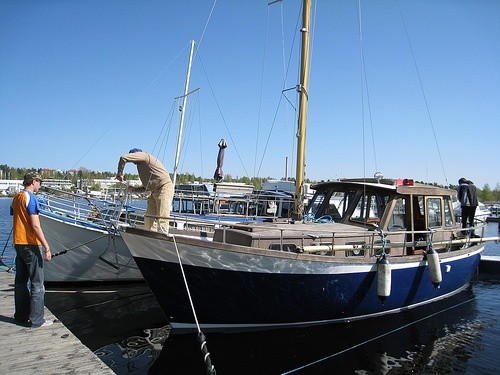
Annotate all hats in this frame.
[24,172,44,182]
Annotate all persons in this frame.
[458,178,478,238]
[115,148,174,235]
[10,172,53,327]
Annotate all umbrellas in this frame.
[212,139,228,212]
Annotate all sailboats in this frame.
[32,0,500,374]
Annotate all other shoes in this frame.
[42,320,54,326]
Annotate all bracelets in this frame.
[44,249,50,253]
